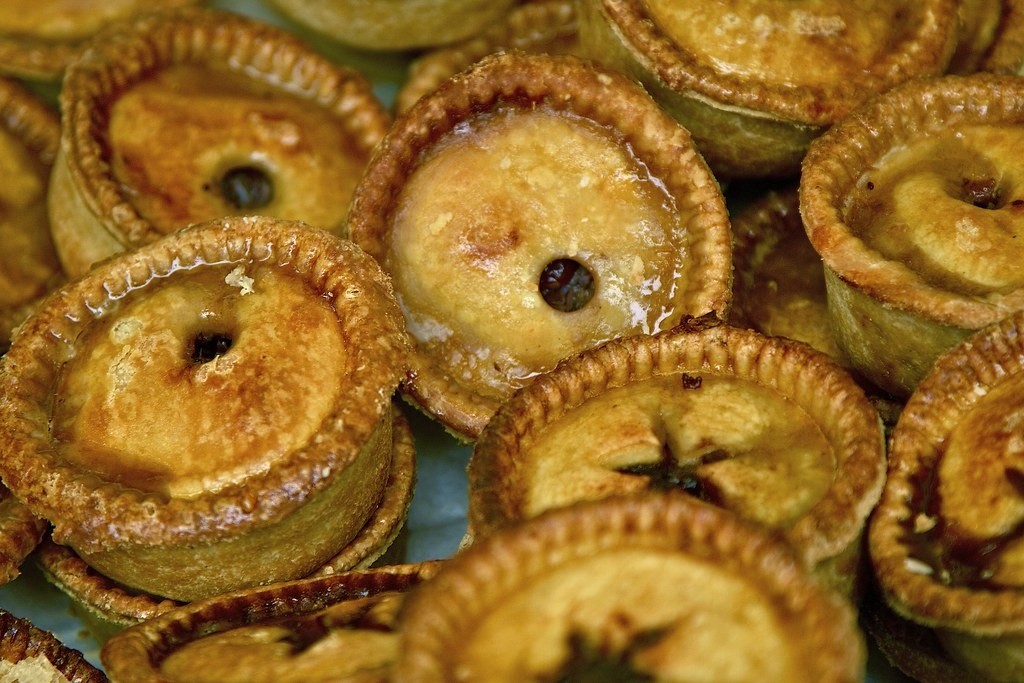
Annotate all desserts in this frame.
[0,0,1024,683]
